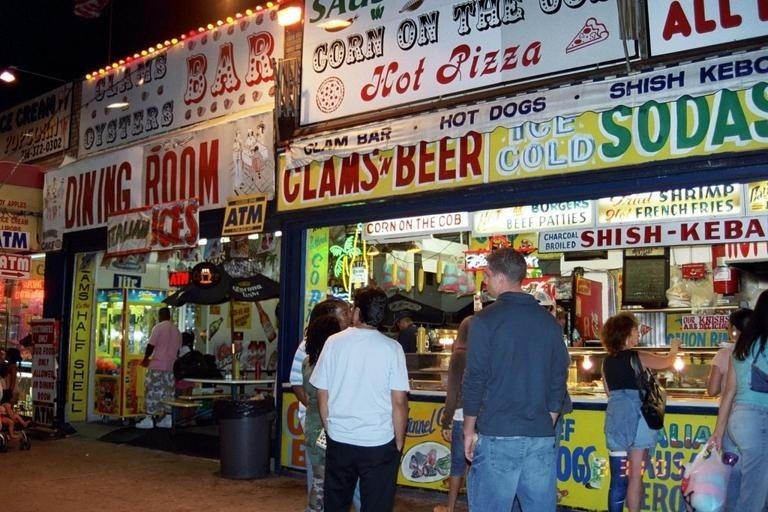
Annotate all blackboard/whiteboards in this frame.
[621,256,670,305]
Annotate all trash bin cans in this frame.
[215,395,276,479]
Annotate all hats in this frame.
[392,311,412,331]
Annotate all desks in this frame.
[182,378,274,405]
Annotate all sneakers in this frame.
[136,415,153,429]
[156,415,172,428]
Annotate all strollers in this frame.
[0,416,33,451]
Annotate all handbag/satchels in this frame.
[316,428,327,450]
[628,350,667,429]
[680,443,738,512]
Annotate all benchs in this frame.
[160,400,202,408]
[179,393,248,411]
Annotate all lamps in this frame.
[580,352,595,370]
[671,356,683,369]
[277,2,305,29]
[108,71,129,110]
[435,327,455,345]
[0,65,71,86]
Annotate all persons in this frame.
[708,306,755,407]
[309,286,409,512]
[434,315,472,511]
[177,331,194,359]
[463,248,569,512]
[136,307,182,428]
[706,289,768,511]
[302,295,353,512]
[601,312,682,512]
[0,345,13,405]
[0,390,31,440]
[290,330,361,512]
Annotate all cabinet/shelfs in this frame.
[274,381,721,512]
